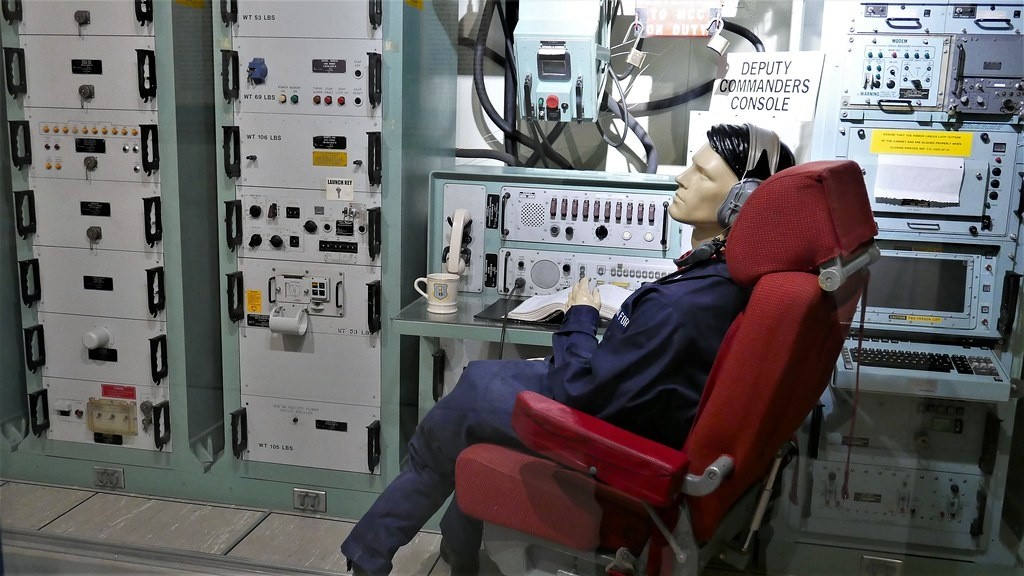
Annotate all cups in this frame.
[414,273,460,313]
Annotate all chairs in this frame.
[456,159,880,576]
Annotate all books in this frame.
[474,284,634,328]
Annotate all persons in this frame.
[341,122,796,575]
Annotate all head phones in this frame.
[718,125,781,233]
